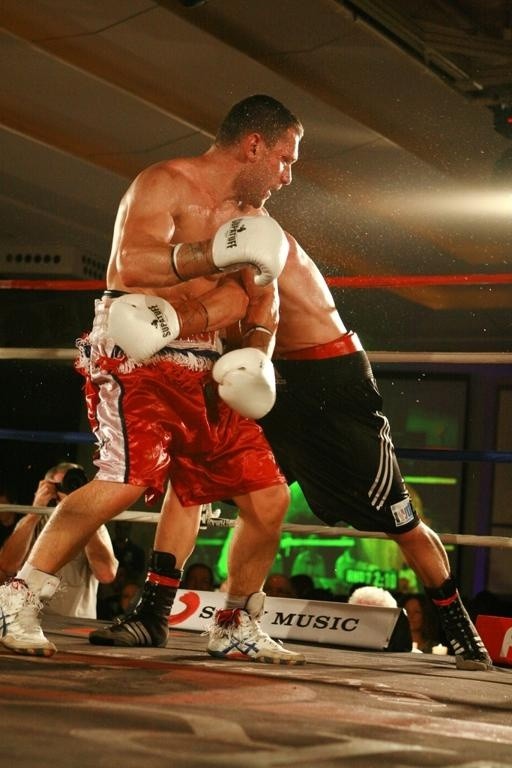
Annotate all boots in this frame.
[88,552,185,647]
[424,575,491,671]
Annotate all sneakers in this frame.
[206,592,305,666]
[0,570,61,655]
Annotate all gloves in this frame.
[213,347,277,419]
[212,215,290,287]
[108,293,181,359]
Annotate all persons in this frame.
[86,229,499,672]
[1,460,512,651]
[2,92,304,664]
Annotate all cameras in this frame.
[54,468,87,495]
